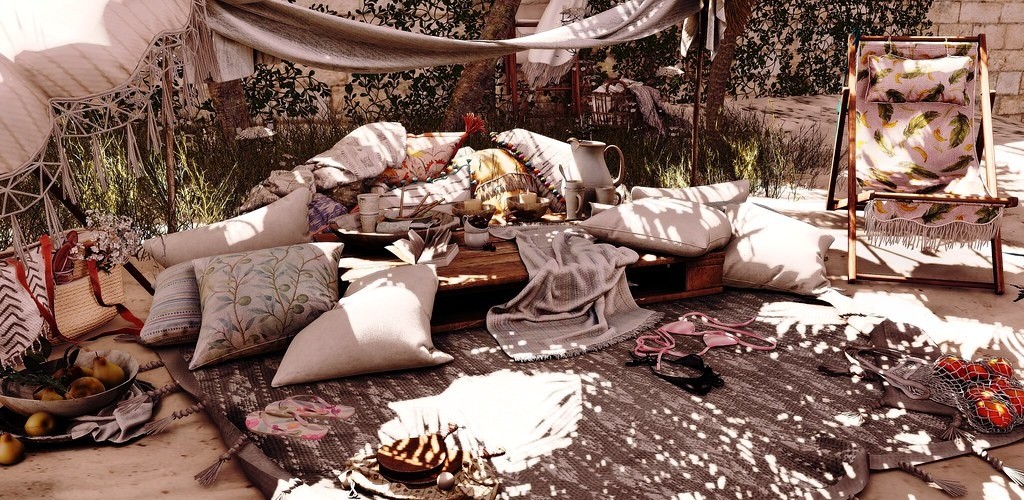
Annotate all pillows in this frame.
[488,124,629,214]
[139,257,203,348]
[718,200,835,298]
[139,186,313,270]
[632,178,750,212]
[186,242,345,371]
[578,198,733,257]
[355,159,473,211]
[365,113,485,187]
[270,263,455,387]
[451,146,566,226]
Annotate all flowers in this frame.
[70,209,146,275]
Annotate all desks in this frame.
[337,226,727,335]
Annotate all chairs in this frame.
[826,31,1020,296]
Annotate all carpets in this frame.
[154,275,1024,500]
